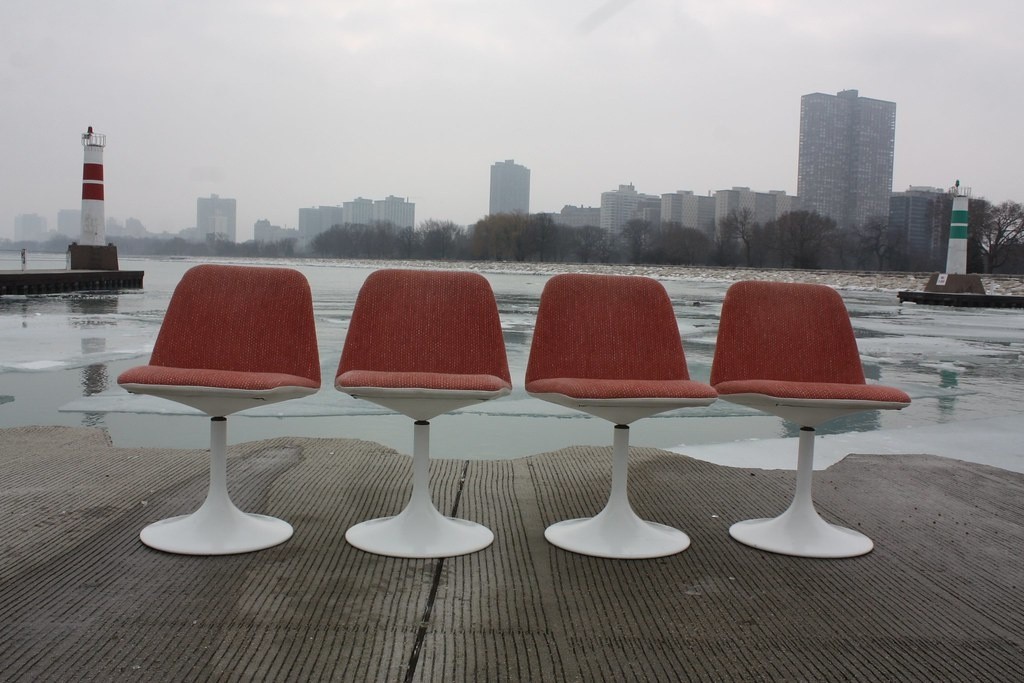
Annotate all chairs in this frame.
[711,279,911,559]
[118,264,321,556]
[524,273,719,560]
[334,269,513,560]
